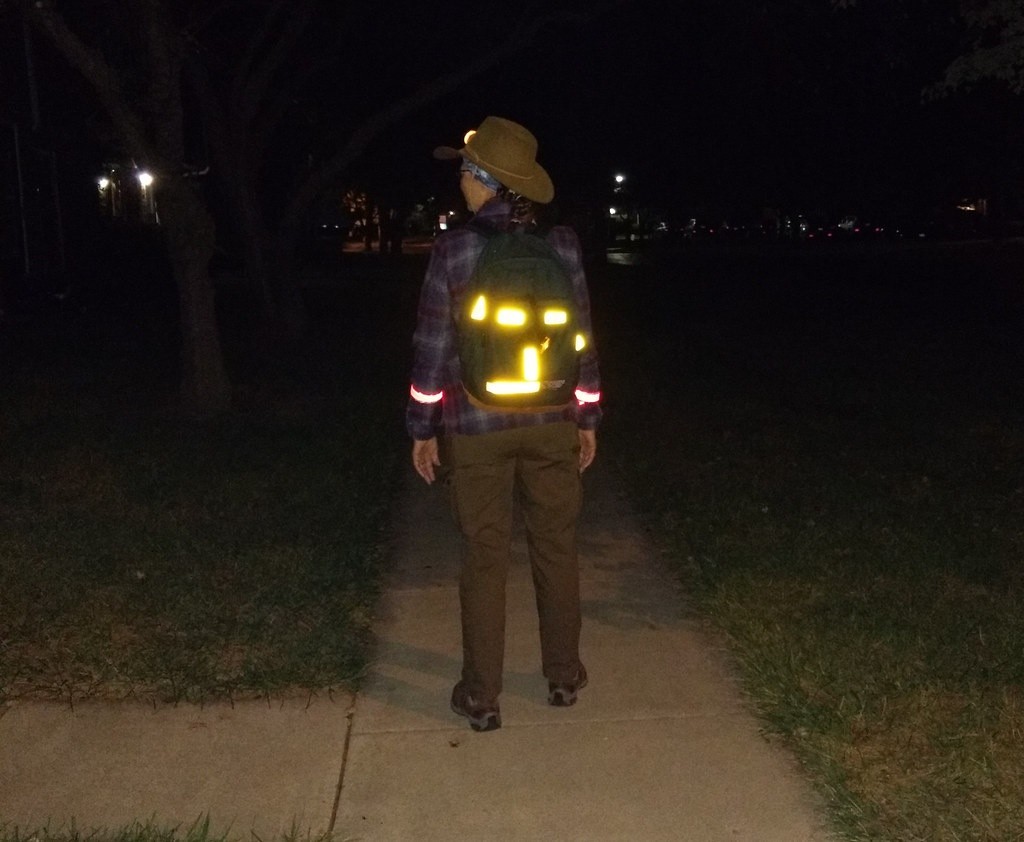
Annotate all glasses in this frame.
[454,168,472,178]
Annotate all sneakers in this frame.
[549,661,588,705]
[450,680,501,732]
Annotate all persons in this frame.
[405,116,602,732]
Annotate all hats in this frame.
[434,116,555,203]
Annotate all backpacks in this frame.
[458,214,581,413]
